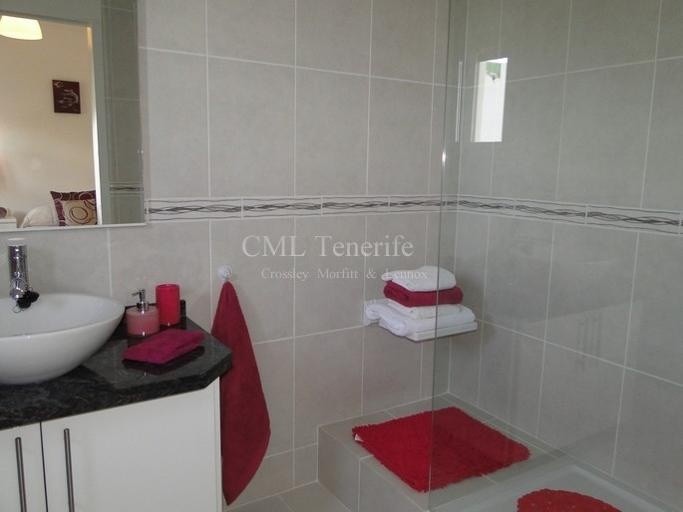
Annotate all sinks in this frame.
[0,294,126,385]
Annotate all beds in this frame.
[0,216,18,229]
[21,206,55,228]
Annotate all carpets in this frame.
[352,405,528,487]
[518,488,620,512]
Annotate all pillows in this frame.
[49,188,99,227]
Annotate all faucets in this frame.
[7,238,28,298]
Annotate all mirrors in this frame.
[0,0,147,231]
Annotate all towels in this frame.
[211,282,270,506]
[124,327,205,368]
[365,267,476,338]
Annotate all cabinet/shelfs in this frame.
[0,380,222,512]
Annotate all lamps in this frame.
[0,16,44,40]
